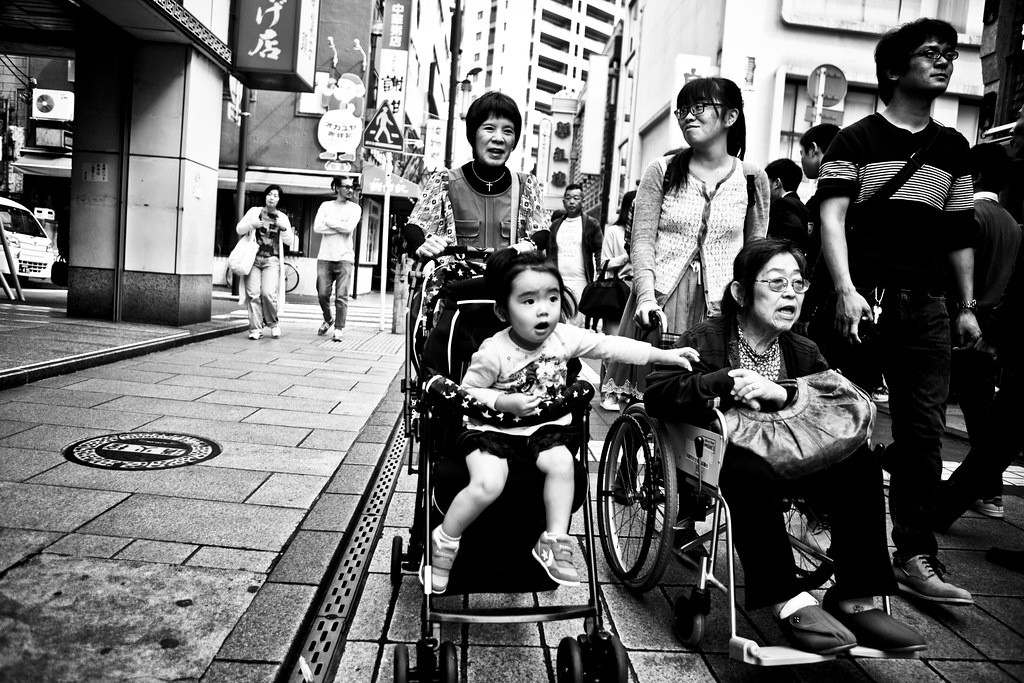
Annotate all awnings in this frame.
[361,162,421,198]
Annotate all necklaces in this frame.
[472,160,506,192]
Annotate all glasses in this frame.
[755,277,811,294]
[341,186,353,189]
[913,50,959,59]
[674,103,719,120]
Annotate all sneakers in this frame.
[419,530,461,595]
[889,554,974,603]
[532,531,581,587]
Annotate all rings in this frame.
[750,383,754,390]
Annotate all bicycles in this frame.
[225,261,300,293]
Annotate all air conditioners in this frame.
[31,88,75,122]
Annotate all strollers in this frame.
[389,244,630,683]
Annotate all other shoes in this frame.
[822,593,929,651]
[318,319,334,335]
[778,591,857,655]
[598,397,622,411]
[977,497,1004,518]
[271,325,280,338]
[248,330,263,339]
[332,329,343,341]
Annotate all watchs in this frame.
[956,298,977,309]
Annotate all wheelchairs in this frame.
[595,308,920,669]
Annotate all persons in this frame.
[314,174,362,341]
[403,95,552,330]
[529,76,1024,536]
[417,258,701,595]
[549,184,603,334]
[643,237,929,654]
[600,189,637,413]
[806,17,982,604]
[227,184,295,339]
[288,226,300,257]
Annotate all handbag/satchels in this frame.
[714,368,870,479]
[228,236,260,275]
[51,254,68,287]
[579,258,630,321]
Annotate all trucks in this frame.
[0,196,55,284]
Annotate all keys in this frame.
[872,301,882,325]
